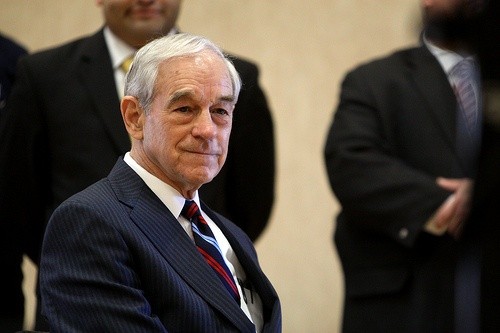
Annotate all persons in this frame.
[1,34,34,332]
[322,0,500,333]
[37,34,282,332]
[12,0,276,264]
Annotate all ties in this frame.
[182,200,241,304]
[451,61,477,135]
